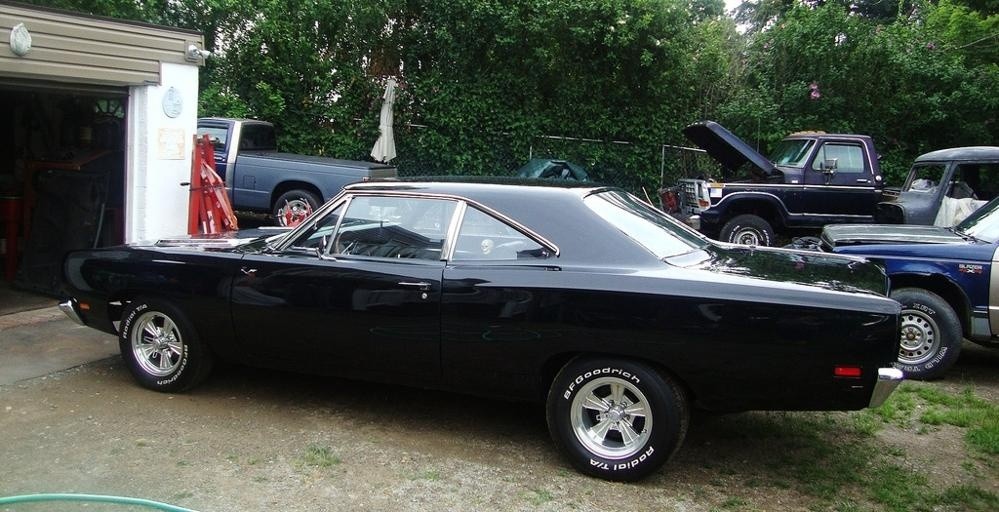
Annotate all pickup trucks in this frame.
[196,117,396,226]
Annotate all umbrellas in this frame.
[370,75,403,165]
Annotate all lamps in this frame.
[187,44,212,62]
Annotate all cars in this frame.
[58,175,902,480]
